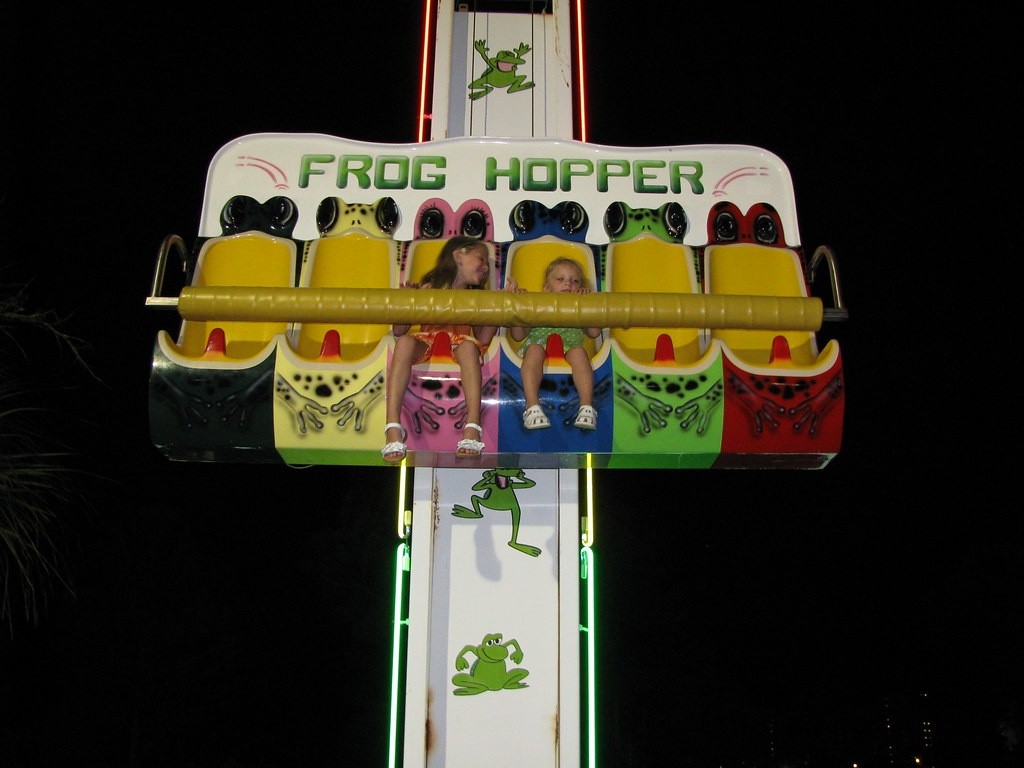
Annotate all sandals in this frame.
[574,405,598,430]
[522,405,551,430]
[381,423,407,462]
[456,422,485,457]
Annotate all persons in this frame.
[381,237,519,463]
[510,256,601,431]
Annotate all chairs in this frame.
[146,195,304,467]
[498,199,613,469]
[273,195,391,466]
[603,202,721,469]
[388,197,499,469]
[700,201,842,473]
